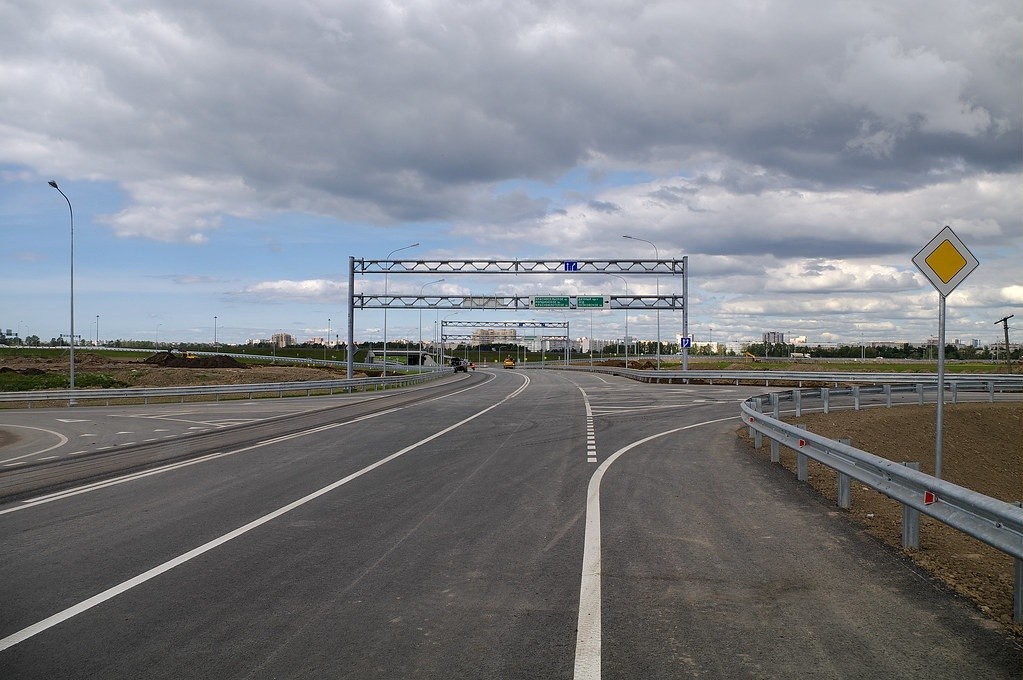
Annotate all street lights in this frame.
[90,315,100,346]
[605,273,628,369]
[709,329,712,355]
[328,318,331,348]
[419,278,445,372]
[442,312,459,365]
[155,324,162,350]
[214,316,217,351]
[17,320,29,346]
[273,329,282,361]
[47,179,75,407]
[623,234,660,372]
[383,243,419,378]
[406,326,421,368]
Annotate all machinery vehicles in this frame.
[745,351,761,363]
[504,357,516,369]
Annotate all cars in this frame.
[468,363,476,370]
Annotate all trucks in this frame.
[452,358,467,372]
[791,352,811,358]
